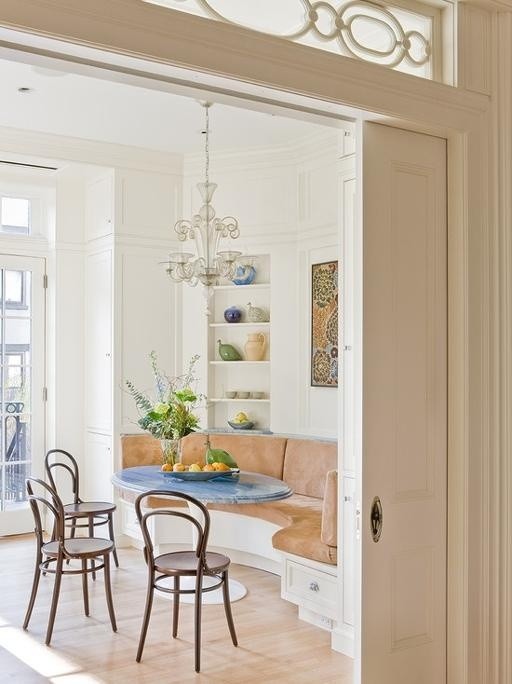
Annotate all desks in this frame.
[111,465,294,604]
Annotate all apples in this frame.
[231,413,248,423]
[162,462,228,472]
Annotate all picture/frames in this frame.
[310,259,338,387]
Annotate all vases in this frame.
[160,438,181,466]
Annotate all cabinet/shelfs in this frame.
[205,254,273,435]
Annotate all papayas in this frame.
[207,449,238,468]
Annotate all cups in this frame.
[244,332,267,361]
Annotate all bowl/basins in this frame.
[227,421,256,430]
[158,465,240,481]
[224,391,264,400]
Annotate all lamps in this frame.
[160,100,260,319]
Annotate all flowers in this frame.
[126,350,207,465]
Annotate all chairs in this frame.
[24,448,119,645]
[135,489,239,673]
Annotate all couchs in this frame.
[121,433,337,632]
